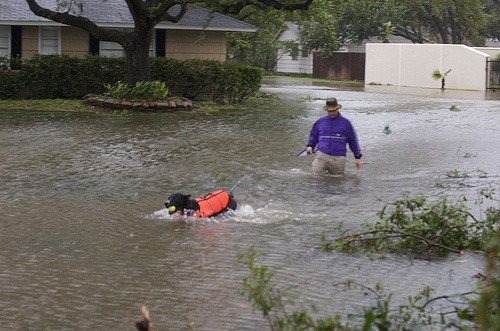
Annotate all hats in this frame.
[322,98,342,111]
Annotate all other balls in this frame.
[168,205,175,214]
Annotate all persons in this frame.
[306,97,362,176]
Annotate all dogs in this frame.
[165,191,237,218]
